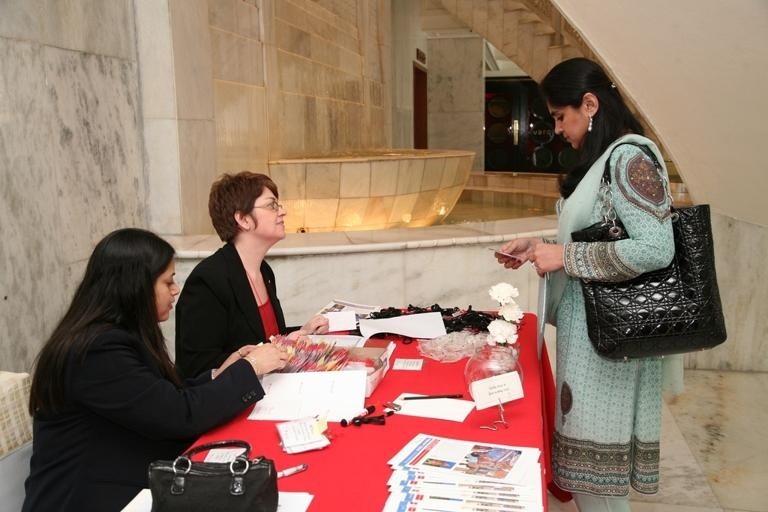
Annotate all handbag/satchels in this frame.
[148,440,278,512]
[571,205,727,359]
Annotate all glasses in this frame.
[253,202,281,210]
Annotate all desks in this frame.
[150,305,572,512]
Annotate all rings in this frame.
[531,262,538,270]
[236,349,242,357]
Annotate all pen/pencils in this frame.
[404,394,463,400]
[276,464,307,479]
[341,406,396,427]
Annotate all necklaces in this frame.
[246,271,263,305]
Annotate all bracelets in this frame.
[243,354,259,376]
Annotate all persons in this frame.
[20,227,291,511]
[465,445,514,479]
[170,168,331,385]
[493,58,677,512]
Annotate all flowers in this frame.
[485,281,524,349]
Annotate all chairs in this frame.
[0,440,34,512]
